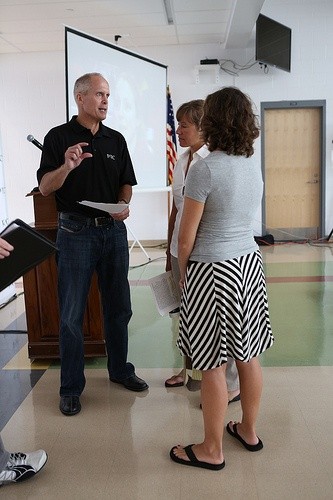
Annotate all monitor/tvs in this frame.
[254,14,292,72]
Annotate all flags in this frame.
[166,85,178,191]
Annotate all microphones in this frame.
[27,135,43,150]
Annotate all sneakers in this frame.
[0,449,48,486]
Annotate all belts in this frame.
[60,213,115,228]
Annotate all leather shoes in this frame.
[60,393,81,415]
[109,372,149,391]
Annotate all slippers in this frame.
[227,421,263,451]
[165,375,184,387]
[170,444,225,470]
[200,393,241,409]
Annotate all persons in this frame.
[164,99,243,409]
[37,73,149,416]
[170,86,275,471]
[0,237,48,486]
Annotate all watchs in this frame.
[118,198,128,204]
